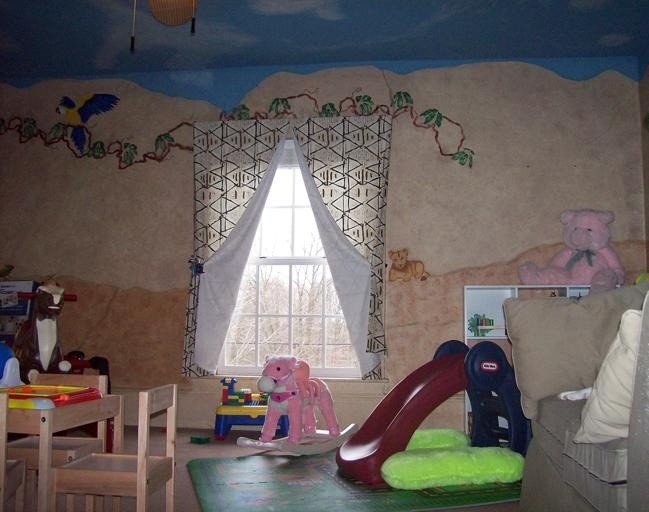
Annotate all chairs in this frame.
[0,370,180,512]
[522,295,649,511]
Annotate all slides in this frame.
[336,353,467,484]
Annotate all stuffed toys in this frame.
[517,207,626,295]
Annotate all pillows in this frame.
[380,427,524,491]
[500,283,642,445]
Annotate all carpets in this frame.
[185,450,524,510]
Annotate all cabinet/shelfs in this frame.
[462,285,621,443]
